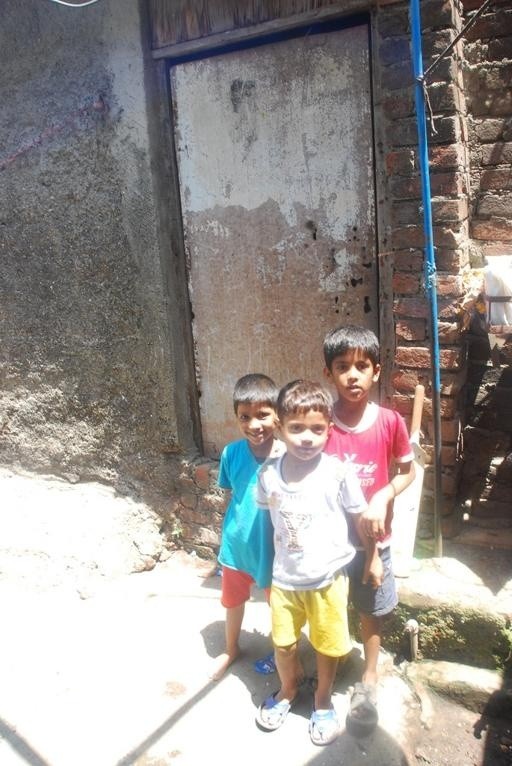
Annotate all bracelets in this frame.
[389,481,399,500]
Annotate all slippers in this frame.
[348,682,378,727]
[309,690,340,745]
[257,686,301,730]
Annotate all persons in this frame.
[253,379,385,747]
[205,372,287,681]
[307,323,418,739]
